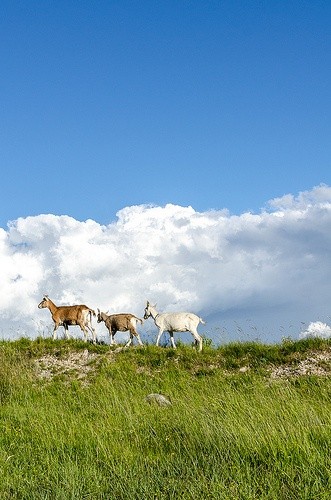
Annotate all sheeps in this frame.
[144,300,205,352]
[97,308,144,347]
[37,295,96,346]
